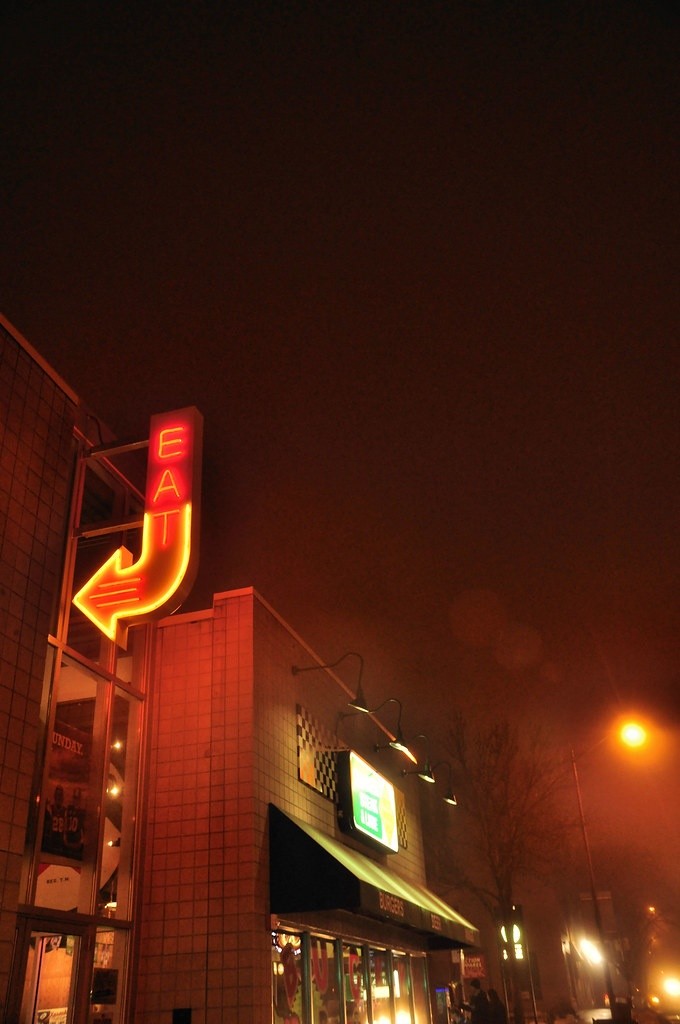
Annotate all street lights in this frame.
[570,716,654,1024]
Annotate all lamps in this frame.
[290,650,456,806]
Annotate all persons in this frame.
[459,978,508,1024]
[40,784,86,860]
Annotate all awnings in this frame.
[268,803,480,951]
